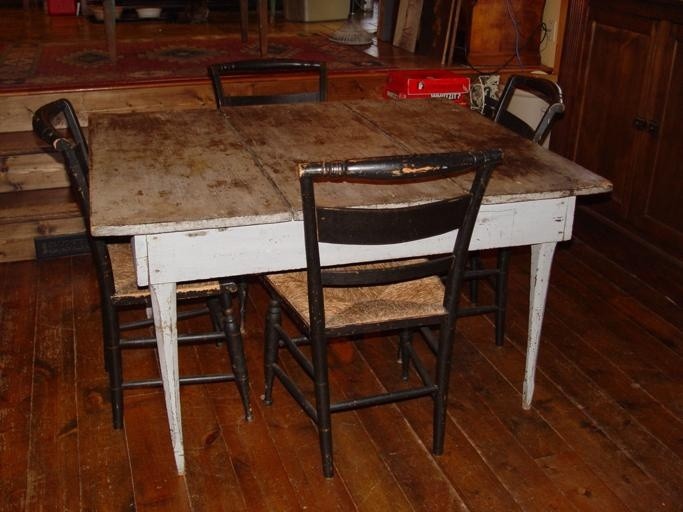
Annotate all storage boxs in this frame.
[380,66,471,109]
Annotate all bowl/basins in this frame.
[136,8,161,19]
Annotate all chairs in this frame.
[393,71,566,367]
[30,95,255,429]
[207,56,331,340]
[254,142,507,482]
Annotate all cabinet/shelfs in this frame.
[419,1,554,76]
[556,0,682,326]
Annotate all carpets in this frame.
[0,27,398,91]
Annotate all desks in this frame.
[101,2,271,67]
